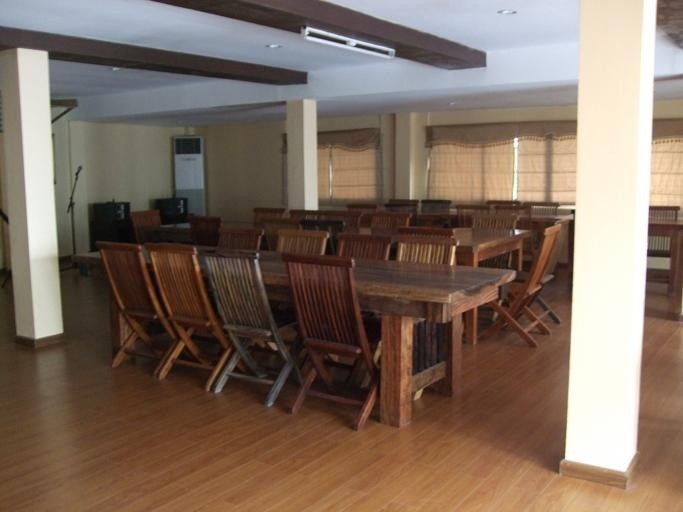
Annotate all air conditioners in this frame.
[173,135,206,216]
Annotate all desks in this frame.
[645,219,683,323]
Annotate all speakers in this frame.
[155,197,188,225]
[92,202,130,252]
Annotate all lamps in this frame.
[302,25,396,60]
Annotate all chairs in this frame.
[647,206,680,280]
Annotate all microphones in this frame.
[75,165,82,176]
